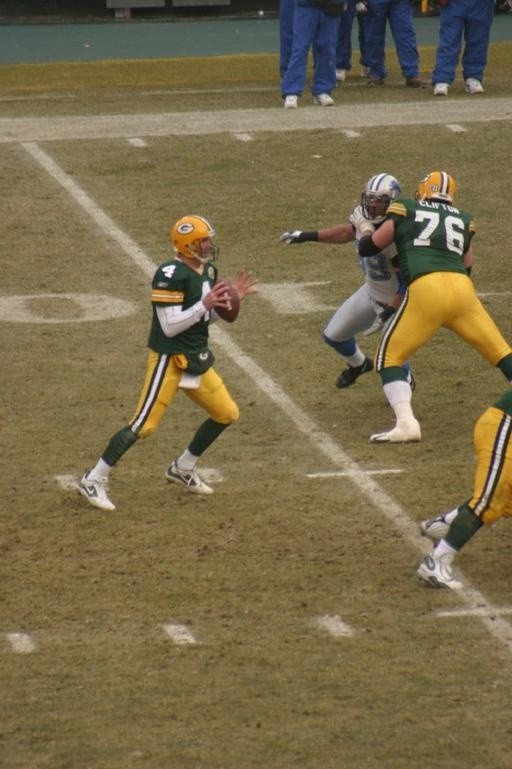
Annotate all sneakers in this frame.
[464,76,484,94]
[311,93,335,106]
[431,81,450,97]
[336,67,347,82]
[366,76,384,88]
[284,93,300,110]
[369,426,422,445]
[334,355,375,389]
[75,470,117,513]
[406,76,427,89]
[415,551,465,591]
[163,459,215,498]
[418,514,453,540]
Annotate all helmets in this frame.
[359,173,402,222]
[170,213,221,266]
[415,169,458,207]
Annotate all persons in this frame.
[278,174,416,401]
[77,214,259,512]
[417,388,511,590]
[348,170,511,444]
[278,0,501,111]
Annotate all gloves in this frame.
[363,299,396,338]
[277,227,304,246]
[348,212,376,236]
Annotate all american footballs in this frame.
[215,277,239,322]
